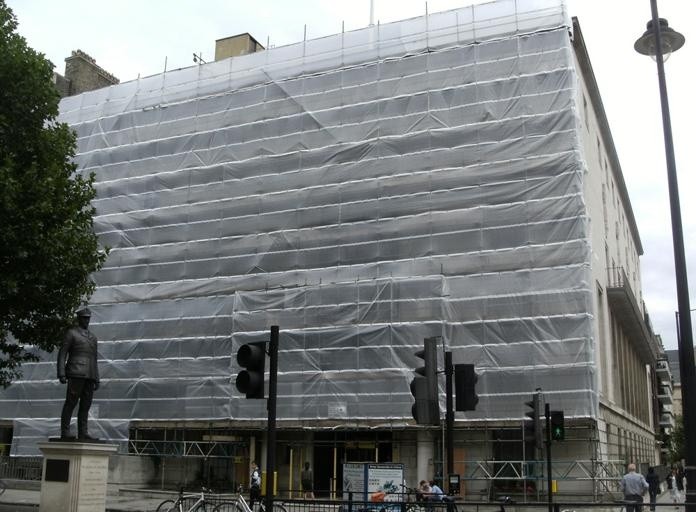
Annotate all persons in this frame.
[646,466,659,512]
[246,461,262,511]
[665,466,684,510]
[300,461,315,500]
[422,480,443,511]
[56,308,101,442]
[414,480,431,507]
[620,464,649,512]
[677,466,683,483]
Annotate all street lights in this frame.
[634,0,696,512]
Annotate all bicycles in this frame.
[156,482,286,512]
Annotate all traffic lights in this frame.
[551,411,564,442]
[410,338,440,426]
[455,364,478,411]
[236,341,266,399]
[524,395,541,449]
